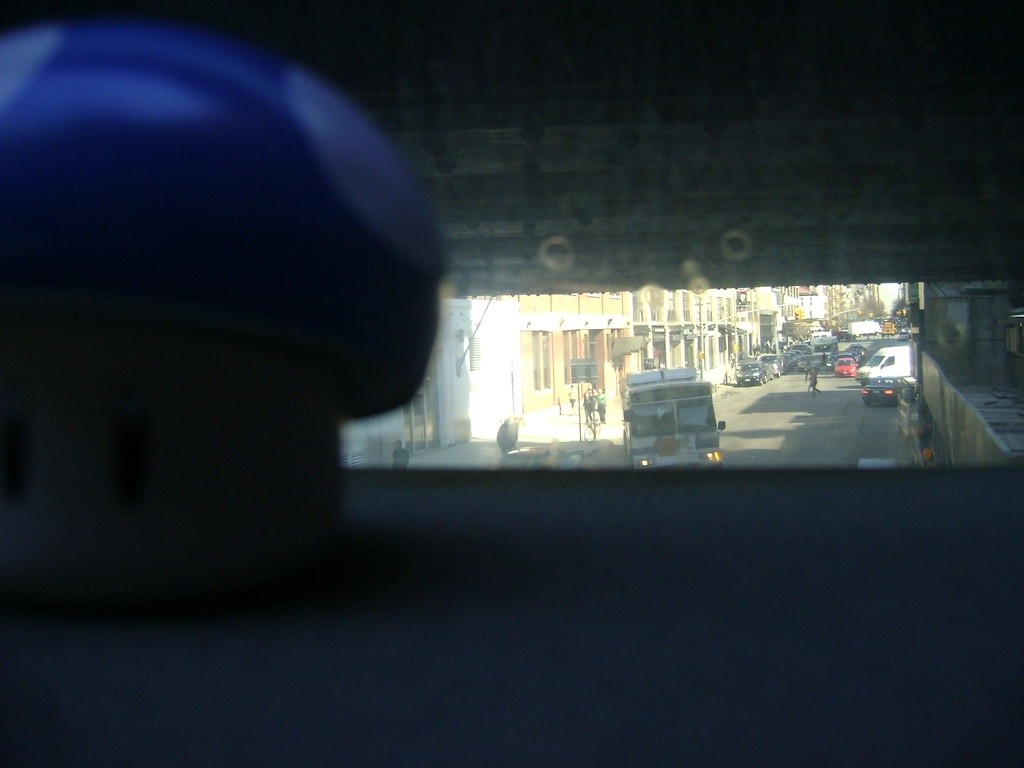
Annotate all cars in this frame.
[734,319,918,409]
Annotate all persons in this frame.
[804,351,827,396]
[729,339,776,368]
[393,440,409,469]
[497,419,518,455]
[568,385,606,424]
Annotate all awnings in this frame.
[611,337,648,357]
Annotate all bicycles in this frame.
[584,417,602,443]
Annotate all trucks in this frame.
[620,365,726,471]
